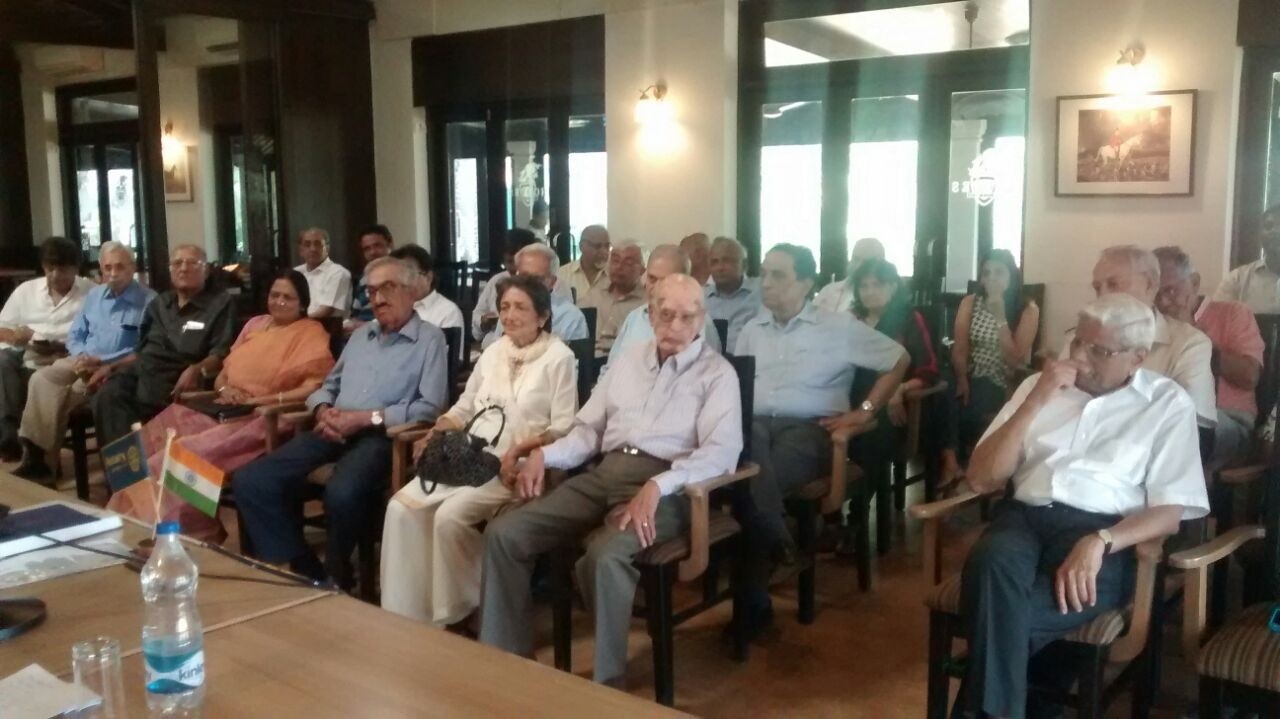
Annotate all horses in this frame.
[1095,135,1142,175]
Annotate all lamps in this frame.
[161,122,179,168]
[1112,48,1144,93]
[634,81,671,129]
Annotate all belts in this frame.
[610,442,658,459]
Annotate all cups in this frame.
[71,635,128,719]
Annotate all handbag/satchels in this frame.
[413,405,504,493]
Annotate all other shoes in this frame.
[9,466,56,488]
[935,468,967,489]
[815,523,847,553]
[781,549,800,562]
[722,612,777,660]
[836,528,857,560]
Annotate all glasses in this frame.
[656,307,697,325]
[170,259,205,270]
[1063,327,1136,359]
[360,283,406,299]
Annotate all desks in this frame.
[0,468,700,719]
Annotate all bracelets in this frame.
[278,391,284,405]
[216,385,230,395]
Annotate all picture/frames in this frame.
[1054,88,1195,197]
[163,146,194,203]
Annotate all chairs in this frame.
[69,279,1280,719]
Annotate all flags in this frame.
[162,441,225,520]
[101,426,150,496]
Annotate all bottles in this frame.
[139,521,205,715]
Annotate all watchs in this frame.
[861,400,876,412]
[370,409,383,427]
[1094,528,1114,555]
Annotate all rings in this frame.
[642,522,647,527]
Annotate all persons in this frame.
[0,198,1279,719]
